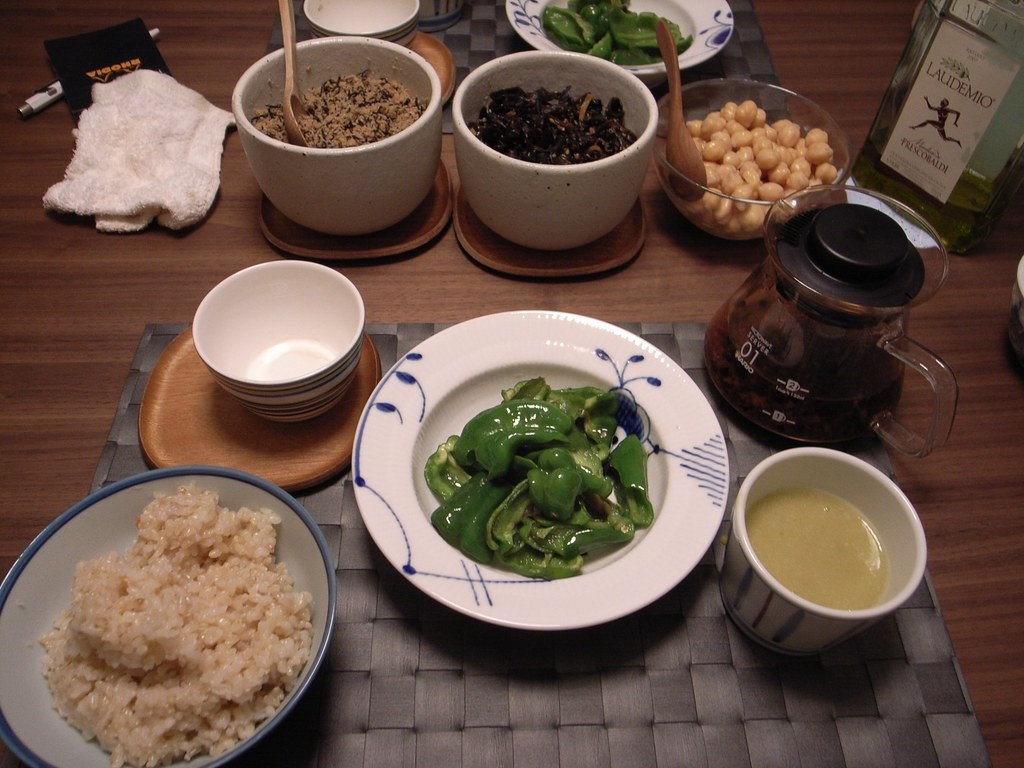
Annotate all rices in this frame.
[36,484,314,768]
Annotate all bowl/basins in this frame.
[418,0,462,32]
[650,78,853,241]
[192,260,366,423]
[0,464,337,768]
[303,0,421,53]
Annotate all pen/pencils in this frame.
[17,27,160,120]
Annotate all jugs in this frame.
[702,183,958,457]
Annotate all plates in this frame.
[255,158,453,260]
[137,327,381,488]
[506,0,734,76]
[453,180,648,279]
[409,30,456,109]
[350,308,732,632]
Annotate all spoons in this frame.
[656,20,707,201]
[278,0,310,148]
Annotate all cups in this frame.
[231,35,443,235]
[720,446,929,656]
[452,48,659,250]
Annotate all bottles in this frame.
[1005,256,1024,366]
[853,0,1024,255]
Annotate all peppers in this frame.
[423,376,656,580]
[540,0,692,67]
